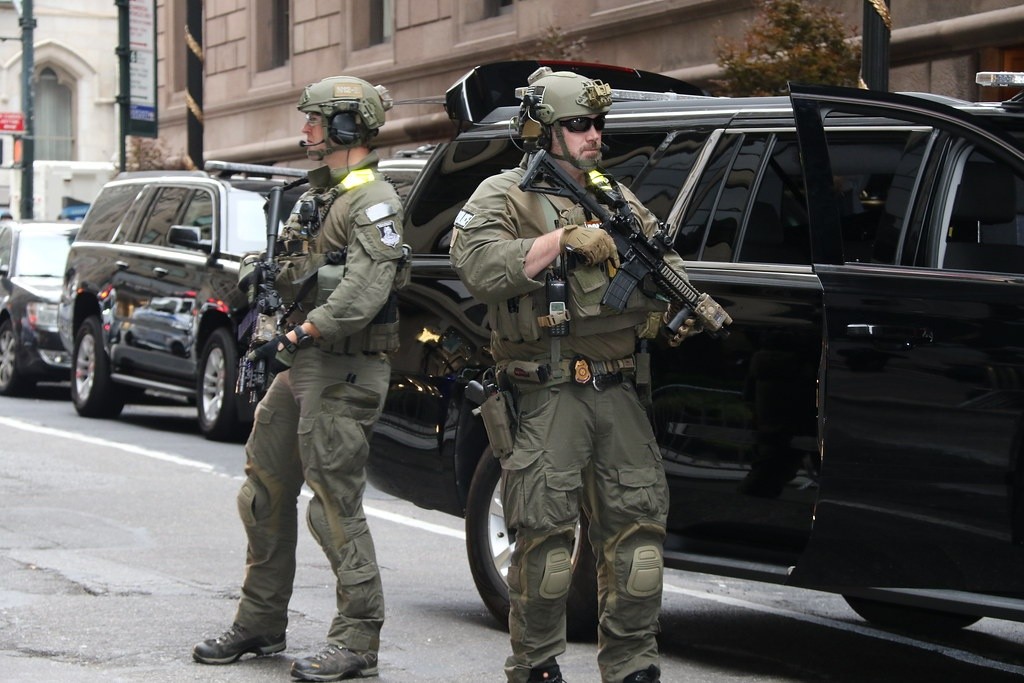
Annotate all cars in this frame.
[0,215,84,398]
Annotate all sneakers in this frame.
[193,622,287,664]
[290,644,378,681]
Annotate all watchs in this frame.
[294,325,315,350]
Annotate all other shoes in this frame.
[622,664,661,683]
[527,664,567,683]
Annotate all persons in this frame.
[449,66,705,683]
[193,76,412,681]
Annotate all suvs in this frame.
[57,141,439,441]
[363,53,1024,660]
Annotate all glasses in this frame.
[551,113,604,131]
[304,114,323,124]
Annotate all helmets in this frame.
[513,66,612,151]
[296,75,393,150]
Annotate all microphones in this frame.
[298,139,325,148]
[600,142,609,153]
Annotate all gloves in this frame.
[670,318,695,346]
[560,223,621,270]
[246,334,298,390]
[248,280,258,302]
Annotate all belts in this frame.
[592,372,620,391]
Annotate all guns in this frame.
[232,185,283,407]
[517,148,734,341]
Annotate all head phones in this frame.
[326,110,360,145]
[516,95,552,155]
